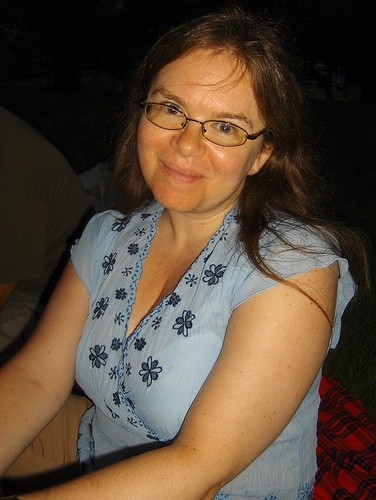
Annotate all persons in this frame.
[2,9,362,500]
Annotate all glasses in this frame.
[144,97,275,146]
